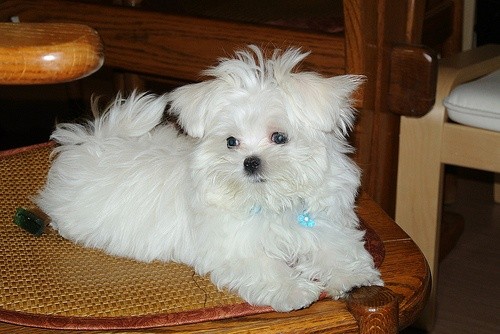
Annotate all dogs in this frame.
[25,43,385,312]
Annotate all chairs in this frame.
[394,1,499,330]
[0,0,436,334]
